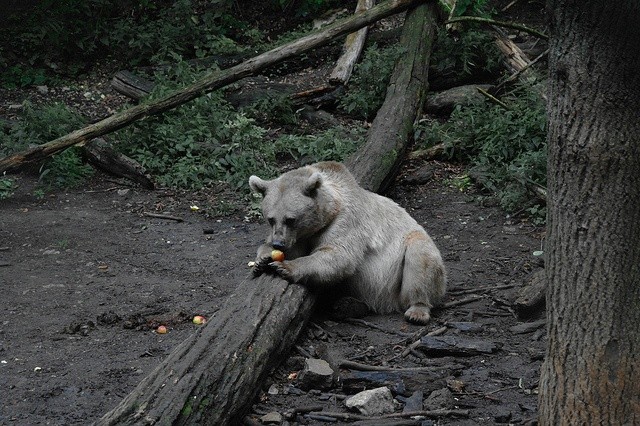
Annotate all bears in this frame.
[249,160,448,324]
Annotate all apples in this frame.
[193,316,207,325]
[271,249,283,260]
